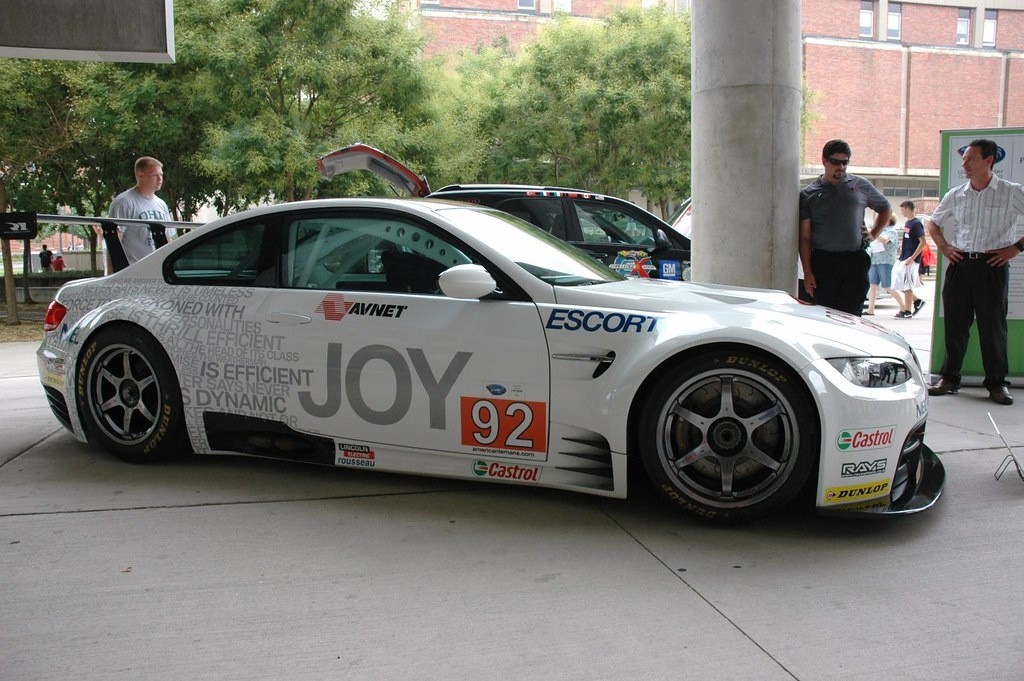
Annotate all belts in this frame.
[958,252,997,259]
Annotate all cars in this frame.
[316,144,693,281]
[35,199,946,529]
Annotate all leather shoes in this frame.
[928,378,961,394]
[990,386,1014,404]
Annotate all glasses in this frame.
[824,156,850,166]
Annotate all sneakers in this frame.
[913,299,926,315]
[903,310,913,317]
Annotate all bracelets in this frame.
[1015,241,1024,252]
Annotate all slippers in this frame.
[894,311,905,317]
[861,312,875,314]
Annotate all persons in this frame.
[39,244,68,273]
[92,156,177,277]
[918,241,936,286]
[918,139,1024,405]
[893,200,926,317]
[799,139,893,317]
[861,214,905,318]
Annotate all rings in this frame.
[999,257,1003,261]
